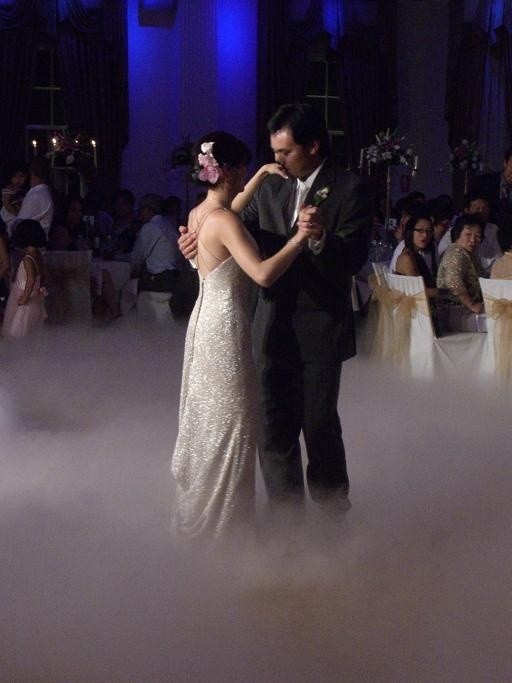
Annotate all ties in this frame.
[290,179,310,228]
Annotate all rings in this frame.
[309,222,314,227]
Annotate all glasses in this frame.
[413,227,433,233]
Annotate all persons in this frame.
[175,101,373,530]
[356,148,512,334]
[0,159,207,338]
[168,130,323,548]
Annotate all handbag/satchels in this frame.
[130,256,147,280]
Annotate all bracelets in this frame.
[288,238,304,255]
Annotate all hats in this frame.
[142,194,163,210]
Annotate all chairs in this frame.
[350,258,512,394]
[0,209,174,327]
